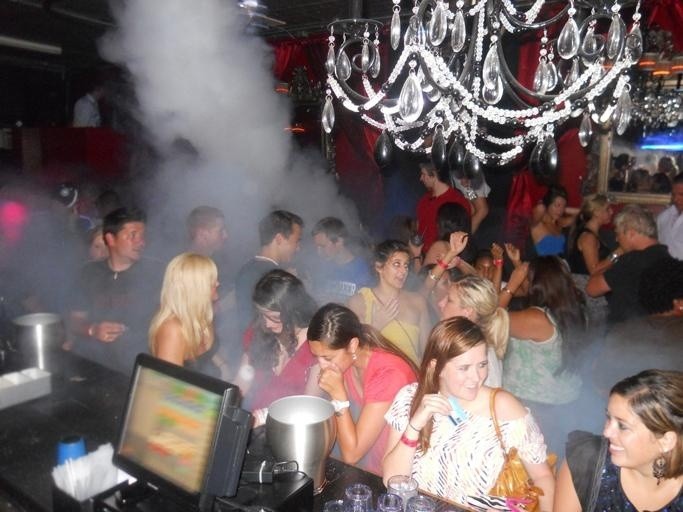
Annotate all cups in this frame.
[380,494,404,511]
[345,484,369,511]
[326,500,350,510]
[389,475,420,499]
[406,496,434,510]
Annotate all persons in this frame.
[87,223,110,261]
[498,256,589,419]
[531,186,579,258]
[609,149,683,194]
[409,202,476,274]
[183,205,228,259]
[452,166,491,233]
[435,274,508,391]
[72,77,106,129]
[231,268,325,430]
[146,251,230,384]
[407,158,472,252]
[63,207,162,376]
[585,205,683,338]
[234,210,306,336]
[654,173,683,262]
[567,190,625,323]
[553,369,683,512]
[306,302,420,479]
[416,231,478,325]
[346,239,431,370]
[51,181,96,230]
[380,315,555,512]
[303,216,376,304]
[472,242,530,297]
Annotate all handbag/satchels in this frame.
[489,387,558,512]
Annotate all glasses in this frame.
[257,310,282,325]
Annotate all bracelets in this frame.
[413,256,421,259]
[607,251,621,264]
[492,259,504,266]
[407,422,422,433]
[438,260,449,269]
[428,269,439,282]
[397,431,418,448]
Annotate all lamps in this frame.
[630,21,683,131]
[321,0,642,184]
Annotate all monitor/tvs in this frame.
[112,353,252,512]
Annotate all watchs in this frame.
[329,399,350,412]
[501,287,514,297]
